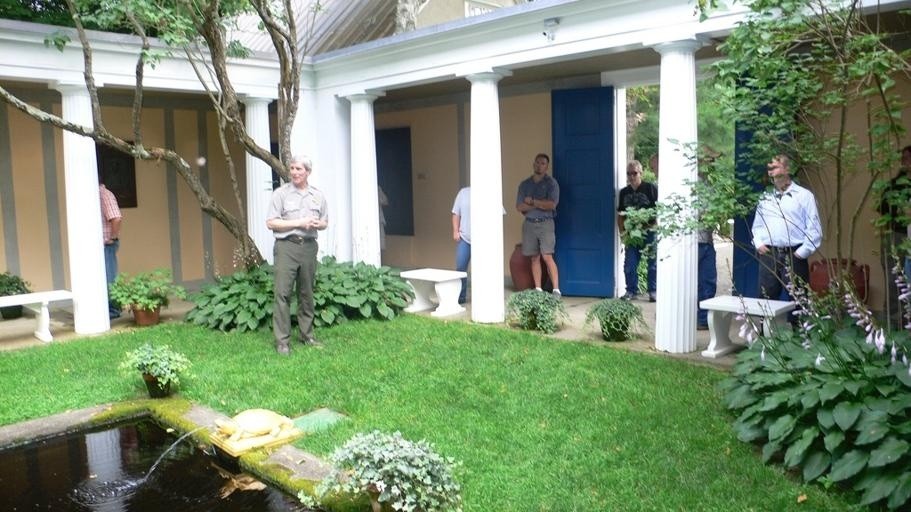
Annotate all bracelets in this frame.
[530,197,535,206]
[111,237,118,241]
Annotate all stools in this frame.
[0,289,75,344]
[398,267,468,319]
[699,295,796,359]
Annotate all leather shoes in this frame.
[297,333,323,346]
[620,293,636,300]
[276,342,290,356]
[649,292,655,301]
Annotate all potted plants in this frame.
[0,270,35,320]
[117,343,195,400]
[106,267,188,327]
[505,287,573,335]
[296,428,466,512]
[583,296,648,343]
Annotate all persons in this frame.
[618,160,657,301]
[378,186,389,266]
[750,155,823,335]
[516,153,562,298]
[650,155,658,187]
[265,158,329,355]
[877,145,911,329]
[697,146,721,330]
[451,186,506,303]
[98,183,122,318]
[896,198,911,302]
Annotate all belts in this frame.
[525,217,553,222]
[763,242,804,254]
[277,235,316,244]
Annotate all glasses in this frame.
[627,171,639,176]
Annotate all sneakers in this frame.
[552,291,561,300]
